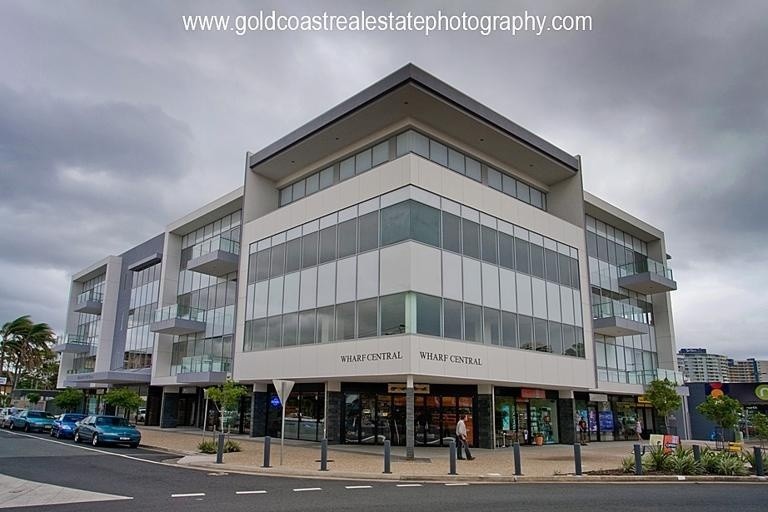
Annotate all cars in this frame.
[0,404,26,431]
[50,412,89,440]
[133,408,146,422]
[73,413,142,449]
[7,409,57,435]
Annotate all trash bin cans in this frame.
[533,433,545,446]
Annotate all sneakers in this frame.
[459,458,465,460]
[469,456,475,460]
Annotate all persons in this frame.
[635,418,642,440]
[456,414,476,460]
[578,416,588,446]
